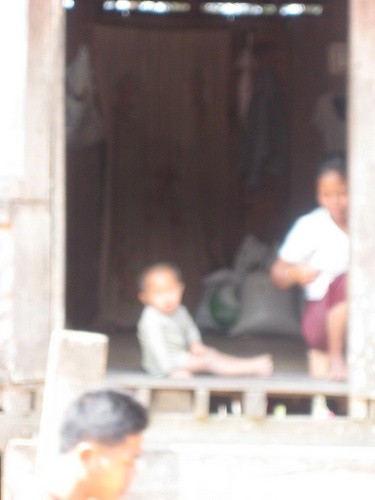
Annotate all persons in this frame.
[271,159,350,417]
[138,261,274,381]
[11,390,149,500]
